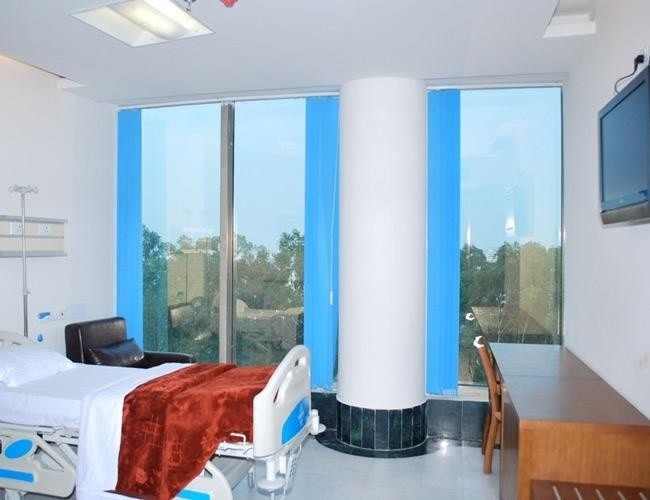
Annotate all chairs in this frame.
[473,335,502,474]
[65,316,196,368]
[168,303,242,359]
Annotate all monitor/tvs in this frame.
[598,65,650,225]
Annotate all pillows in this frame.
[0,342,74,387]
[88,337,145,367]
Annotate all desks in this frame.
[490,341,650,500]
[529,480,650,500]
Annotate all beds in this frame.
[209,298,304,350]
[0,331,323,500]
